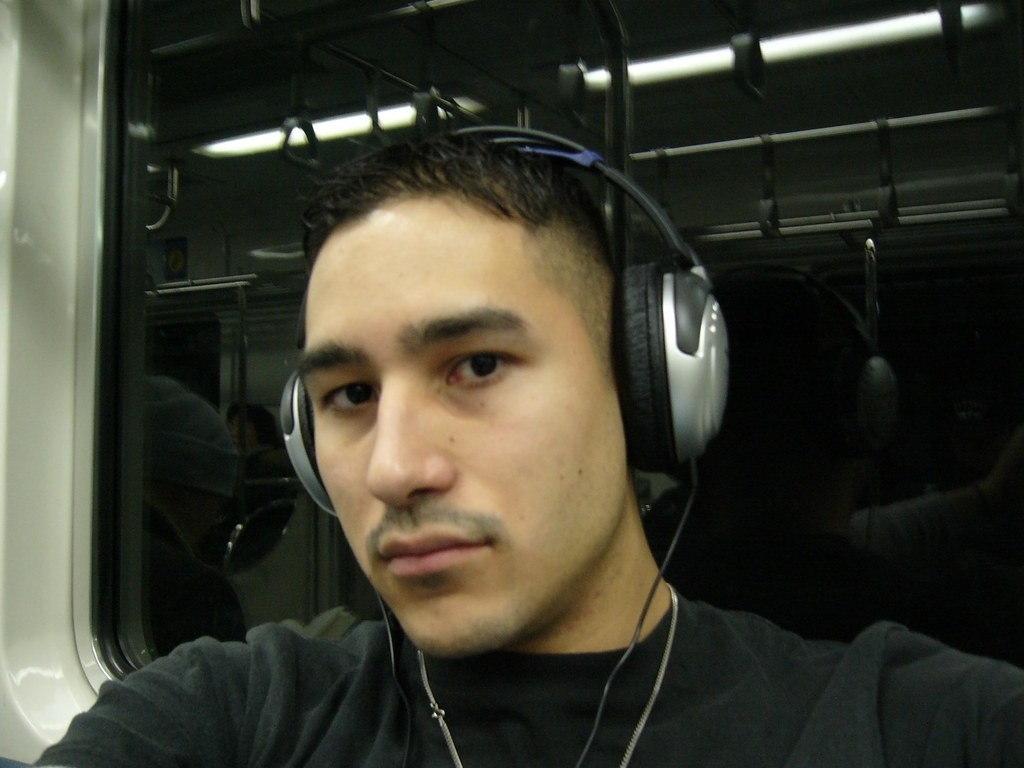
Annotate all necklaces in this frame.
[416,581,680,768]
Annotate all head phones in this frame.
[721,261,899,448]
[281,124,728,516]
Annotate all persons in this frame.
[194,399,300,570]
[34,125,1024,768]
[639,261,1023,672]
